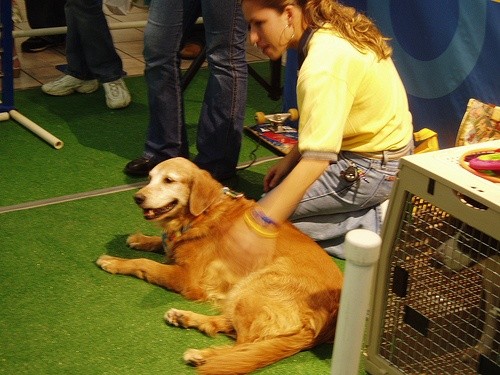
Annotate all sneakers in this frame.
[40,75,98,95]
[102,79,132,109]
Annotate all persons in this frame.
[125,0,250,189]
[241,0,415,260]
[20,0,68,52]
[41,0,132,109]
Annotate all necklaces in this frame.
[296,26,312,79]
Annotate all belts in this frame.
[352,141,414,161]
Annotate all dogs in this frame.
[92,153,344,375]
[425,198,500,364]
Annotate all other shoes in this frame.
[123,156,160,179]
[180,40,204,58]
[22,37,51,51]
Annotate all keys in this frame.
[360,175,369,184]
